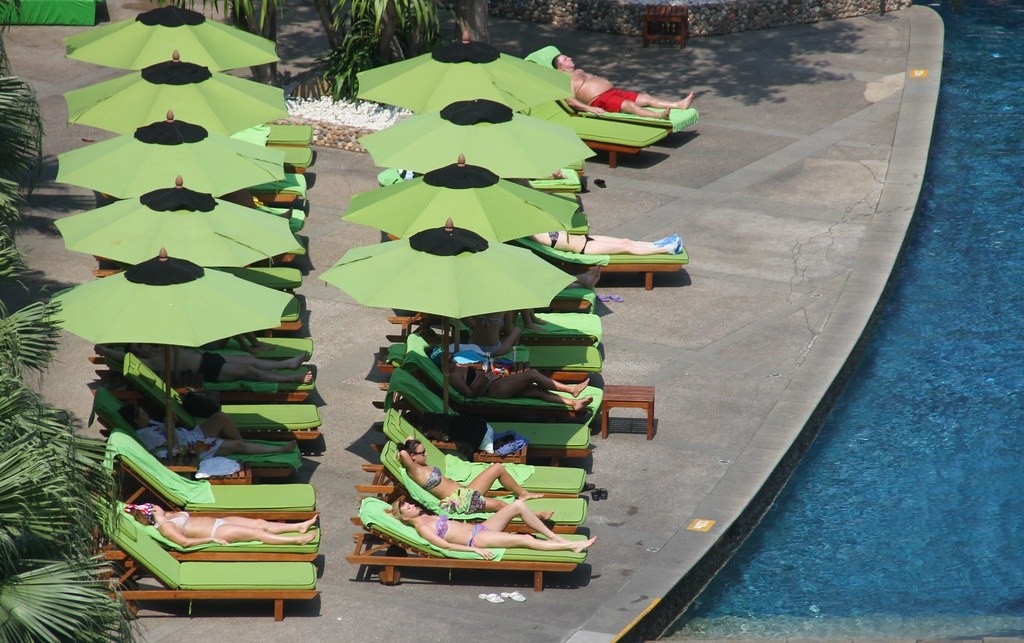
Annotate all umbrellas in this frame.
[323,220,579,437]
[47,6,600,262]
[44,248,295,465]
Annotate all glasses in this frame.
[412,449,425,455]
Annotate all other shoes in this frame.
[600,487,607,500]
[594,179,606,188]
[592,488,599,500]
[581,481,596,492]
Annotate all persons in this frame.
[396,436,555,521]
[126,501,319,548]
[553,54,696,119]
[526,230,682,256]
[463,266,604,358]
[118,404,300,462]
[94,341,313,385]
[386,496,599,561]
[437,352,594,414]
[201,332,279,355]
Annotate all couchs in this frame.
[85,47,700,621]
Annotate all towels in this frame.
[444,453,535,490]
[114,500,263,553]
[101,429,215,504]
[513,237,611,268]
[400,464,525,522]
[450,312,602,346]
[401,333,604,421]
[229,123,270,146]
[250,206,307,231]
[379,168,581,191]
[386,343,528,368]
[554,288,597,314]
[526,46,699,131]
[383,368,460,418]
[87,386,303,470]
[250,172,308,203]
[357,497,506,562]
[190,348,279,393]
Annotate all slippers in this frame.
[608,295,625,302]
[478,593,504,604]
[597,294,610,301]
[500,591,526,601]
